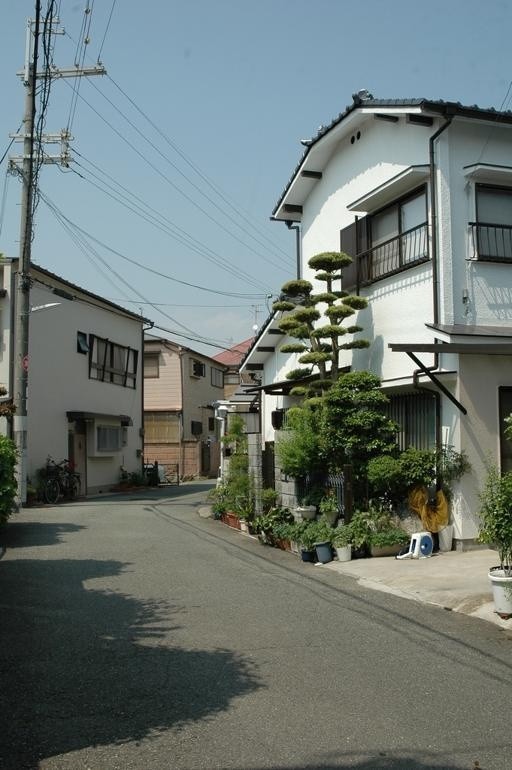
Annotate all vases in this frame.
[438,525,453,552]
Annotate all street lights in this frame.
[11,301,62,507]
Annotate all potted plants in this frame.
[366,498,411,556]
[304,520,332,563]
[27,484,37,507]
[128,472,137,488]
[475,449,512,618]
[349,509,366,557]
[209,472,299,554]
[120,465,128,492]
[331,526,354,562]
[300,524,316,562]
[299,478,326,521]
[318,494,339,524]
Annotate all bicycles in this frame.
[42,455,81,504]
